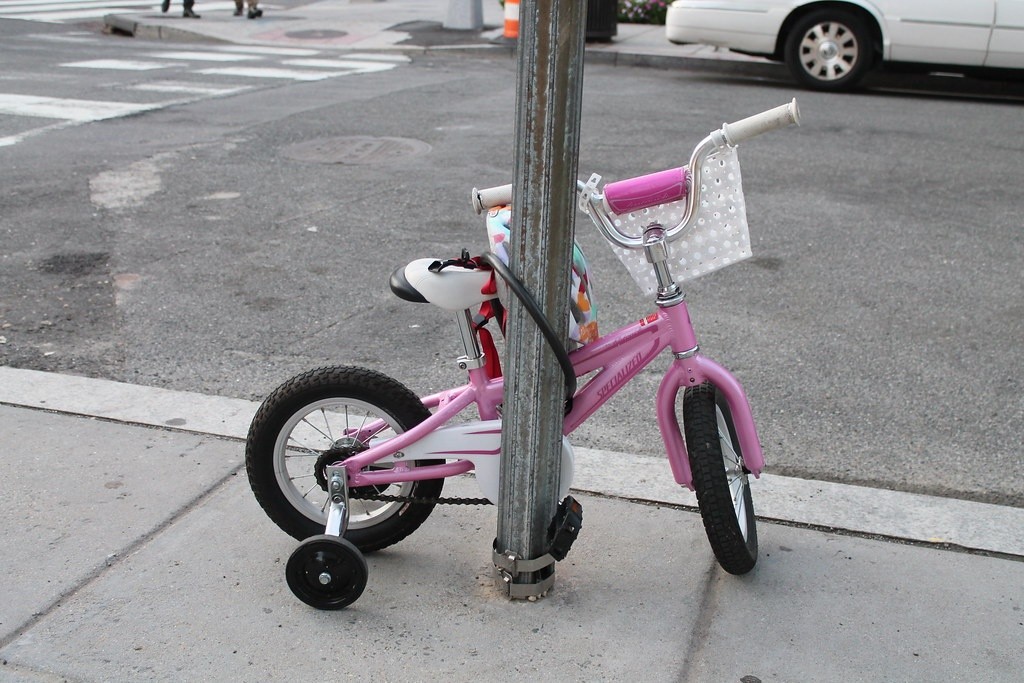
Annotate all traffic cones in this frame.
[504,0,524,40]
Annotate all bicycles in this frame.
[241,96,795,610]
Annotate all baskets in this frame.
[605,142,753,295]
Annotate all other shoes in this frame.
[183,8,200,18]
[235,8,242,16]
[162,0,170,12]
[249,9,263,18]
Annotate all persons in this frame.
[161,0,200,18]
[233,0,262,19]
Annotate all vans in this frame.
[662,0,1023,89]
